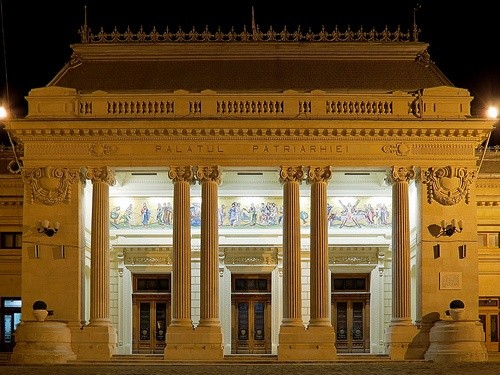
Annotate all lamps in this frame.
[36,219,60,237]
[440,218,463,237]
